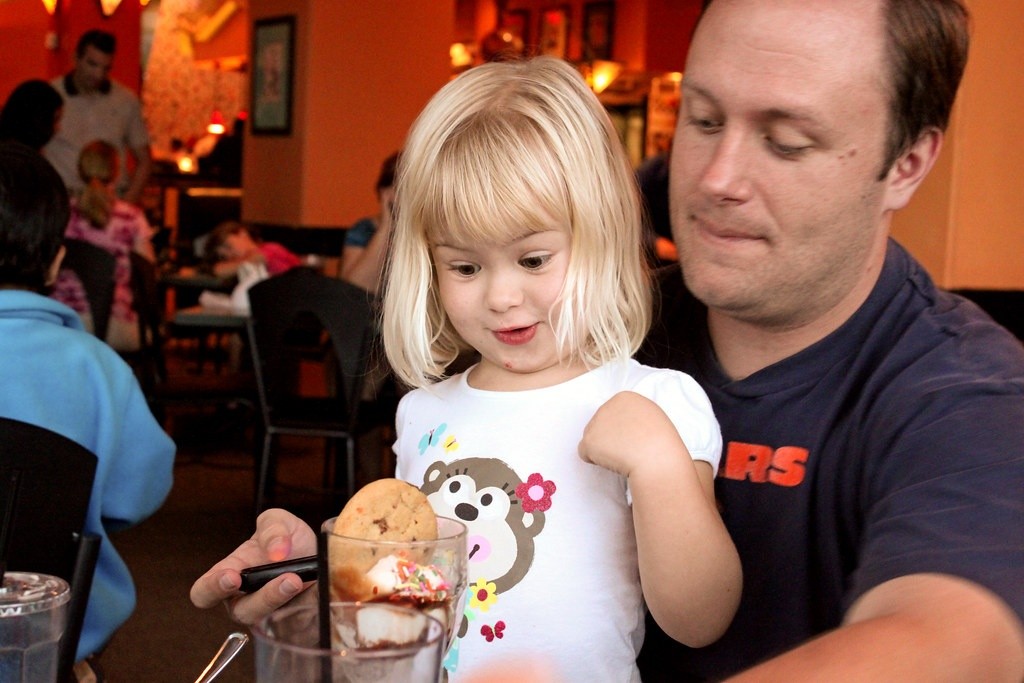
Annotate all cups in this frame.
[322,513,469,659]
[0,571,72,683]
[252,601,444,682]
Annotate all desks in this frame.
[599,90,648,160]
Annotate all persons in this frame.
[43,29,155,201]
[0,79,175,666]
[381,54,741,683]
[180,221,303,279]
[304,148,404,395]
[190,1,1024,683]
[633,153,679,265]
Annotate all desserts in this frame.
[328,554,458,683]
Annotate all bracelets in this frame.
[308,254,317,267]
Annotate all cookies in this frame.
[327,477,439,605]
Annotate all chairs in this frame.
[0,417,103,683]
[129,227,248,394]
[245,278,354,519]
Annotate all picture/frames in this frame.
[250,13,296,136]
[497,7,531,58]
[581,0,616,63]
[536,4,573,59]
